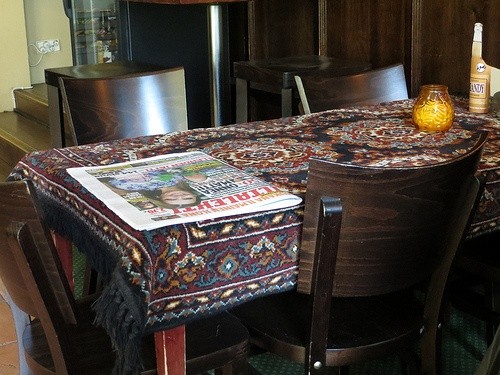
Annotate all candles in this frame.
[412,84,455,132]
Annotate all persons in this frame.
[107,170,201,209]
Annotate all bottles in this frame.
[468,22,491,114]
[96,8,118,65]
[412,84,455,134]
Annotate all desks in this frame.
[233,55,371,125]
[44,59,165,149]
[4,93,500,375]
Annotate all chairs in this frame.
[58,66,189,148]
[0,179,253,375]
[227,130,490,375]
[293,63,409,115]
